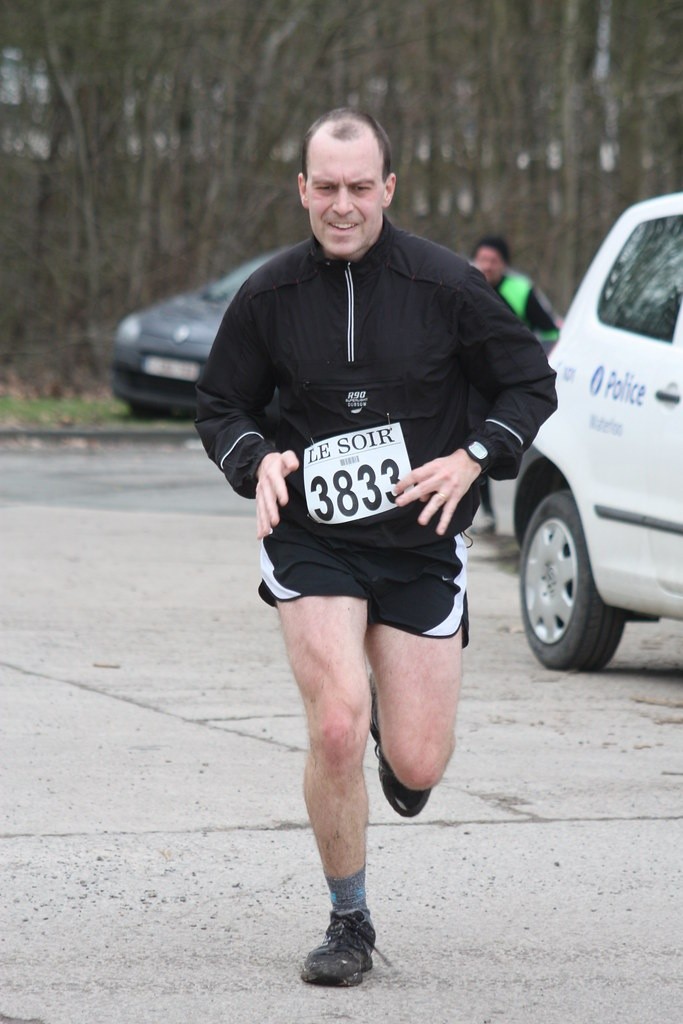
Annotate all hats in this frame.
[470,234,510,268]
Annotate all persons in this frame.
[193,107,558,987]
[471,237,558,536]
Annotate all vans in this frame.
[512,192,683,677]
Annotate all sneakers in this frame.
[368,671,432,817]
[300,908,392,986]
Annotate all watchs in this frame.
[459,440,489,476]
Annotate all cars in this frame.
[106,251,282,423]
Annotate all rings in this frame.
[437,493,447,500]
